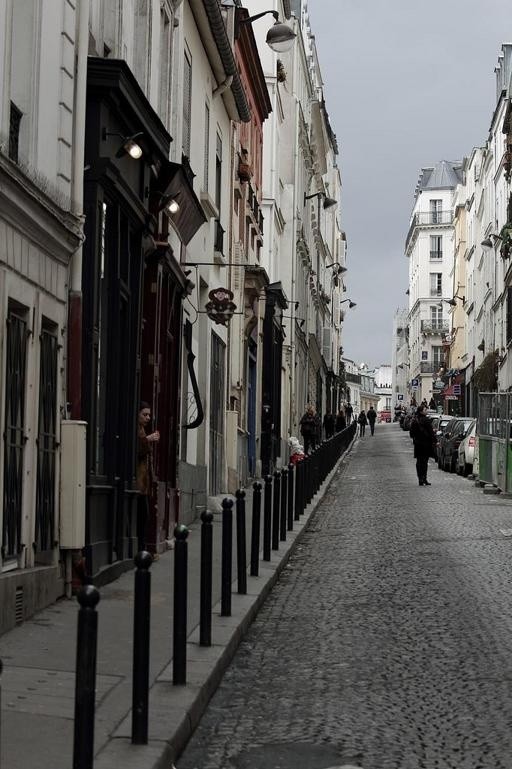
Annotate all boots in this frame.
[416,463,431,485]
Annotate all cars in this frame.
[396,400,512,479]
[380,410,391,420]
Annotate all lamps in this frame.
[234,9,297,52]
[144,186,181,215]
[304,191,338,213]
[447,296,466,307]
[326,262,348,277]
[481,233,504,249]
[102,126,143,161]
[340,299,357,310]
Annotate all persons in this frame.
[392,396,437,416]
[409,405,437,486]
[138,396,164,560]
[299,401,378,455]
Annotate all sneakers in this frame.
[151,552,159,561]
